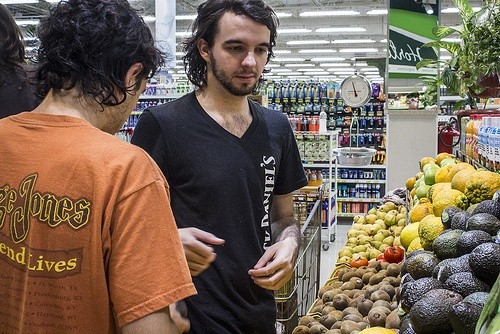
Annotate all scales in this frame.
[333,70,377,167]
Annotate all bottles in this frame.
[355,183,380,199]
[113,82,191,142]
[286,114,320,132]
[464,113,500,173]
[306,196,336,229]
[305,169,323,187]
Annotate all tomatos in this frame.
[350,257,368,267]
[384,246,405,263]
[376,253,388,263]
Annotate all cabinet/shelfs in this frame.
[290,130,338,244]
[439,84,471,148]
[113,94,386,217]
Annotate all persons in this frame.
[130,0,307,334]
[0,0,198,334]
[0,6,34,118]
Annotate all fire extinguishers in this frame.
[438,116,461,153]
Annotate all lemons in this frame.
[400,152,500,255]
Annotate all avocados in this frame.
[398,191,500,334]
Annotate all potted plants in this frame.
[415,0,500,111]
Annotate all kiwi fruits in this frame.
[290,258,402,334]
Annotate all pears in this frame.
[333,202,407,277]
